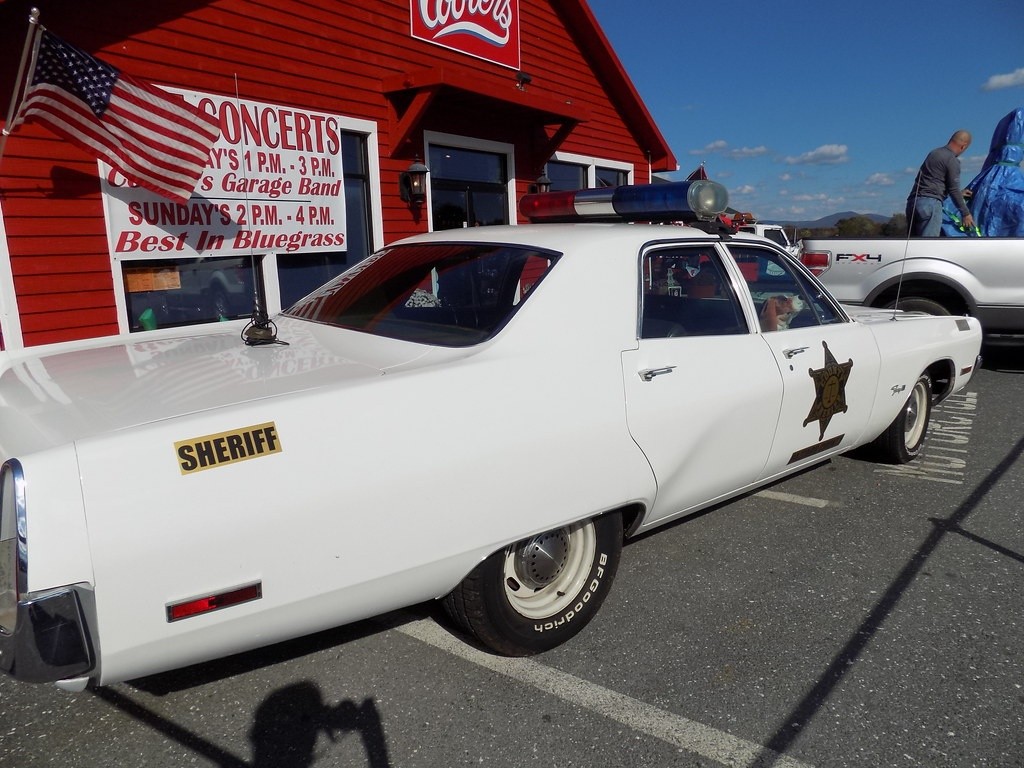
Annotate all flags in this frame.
[8,24,221,206]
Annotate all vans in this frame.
[738,224,801,275]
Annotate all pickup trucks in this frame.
[789,238,1024,350]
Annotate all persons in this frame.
[906,130,976,238]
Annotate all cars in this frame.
[0,175,991,700]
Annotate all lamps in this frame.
[532,169,553,194]
[399,154,431,210]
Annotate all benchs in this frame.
[642,294,823,338]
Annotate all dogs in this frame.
[758,295,805,334]
